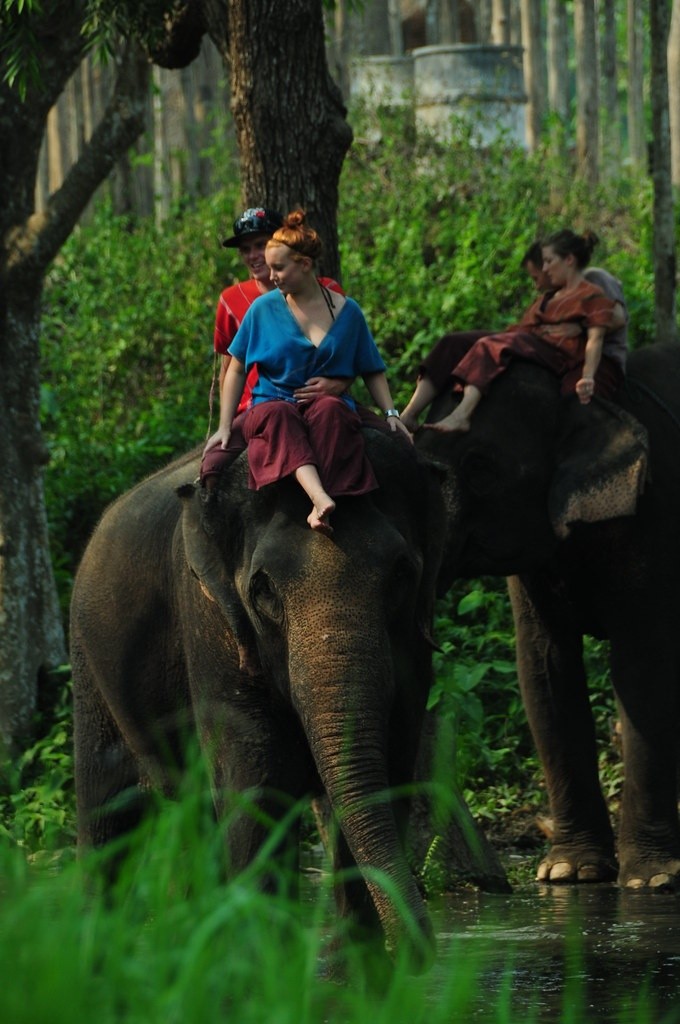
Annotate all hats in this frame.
[223,207,284,248]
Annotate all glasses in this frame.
[233,216,279,235]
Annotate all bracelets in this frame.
[382,408,401,421]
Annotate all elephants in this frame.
[70,332,680,998]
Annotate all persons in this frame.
[197,206,415,535]
[399,228,632,435]
